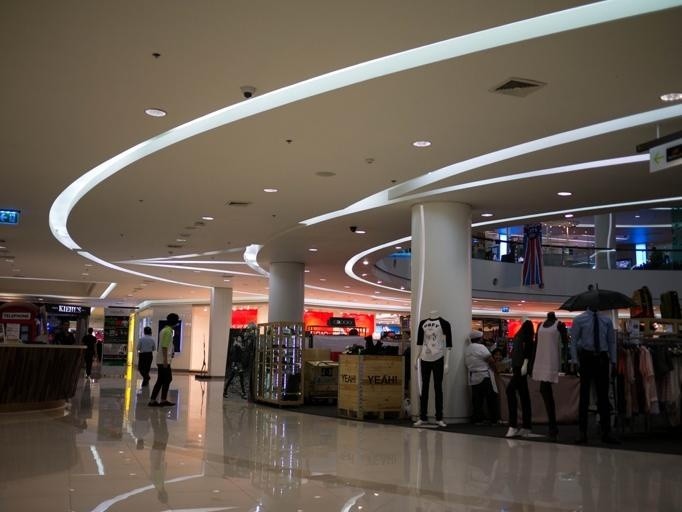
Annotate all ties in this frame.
[592,314,602,351]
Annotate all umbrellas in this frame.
[558,283,639,311]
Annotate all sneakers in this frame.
[140,377,176,407]
[223,393,247,399]
[505,427,532,438]
[412,420,447,428]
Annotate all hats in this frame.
[469,330,483,339]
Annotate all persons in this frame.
[492,348,502,362]
[463,329,507,425]
[54,319,103,377]
[136,326,156,387]
[579,454,611,511]
[528,312,570,437]
[150,405,169,504]
[223,332,253,398]
[147,313,179,406]
[415,429,446,510]
[505,439,536,511]
[567,305,618,441]
[78,378,93,429]
[132,388,151,450]
[505,314,533,438]
[414,308,453,428]
